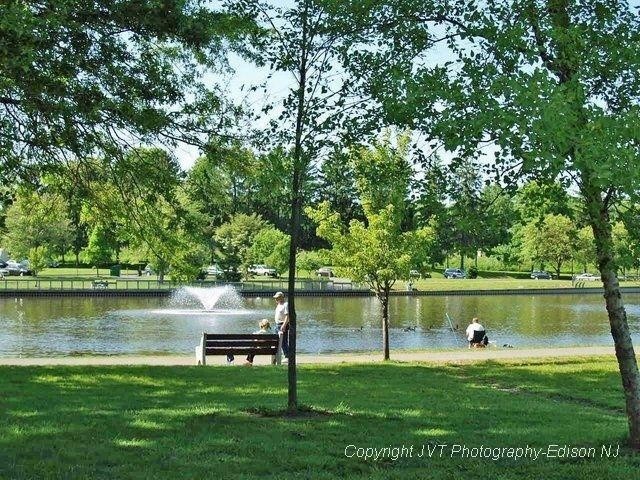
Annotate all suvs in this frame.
[200,264,243,278]
[247,264,277,276]
[145,263,169,276]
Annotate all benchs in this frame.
[194,330,285,366]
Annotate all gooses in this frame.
[352,326,363,332]
[450,324,459,331]
[401,327,410,332]
[410,326,417,331]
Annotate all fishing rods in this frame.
[445,313,459,349]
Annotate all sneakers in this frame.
[282,358,288,363]
[243,361,252,366]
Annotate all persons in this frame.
[466,318,484,341]
[243,319,277,365]
[273,292,289,363]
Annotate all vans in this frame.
[444,268,466,279]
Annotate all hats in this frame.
[273,291,285,299]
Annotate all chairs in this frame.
[470,329,488,349]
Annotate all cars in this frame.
[530,271,550,279]
[574,273,602,282]
[49,261,60,268]
[410,270,430,278]
[0,259,33,277]
[315,265,333,276]
